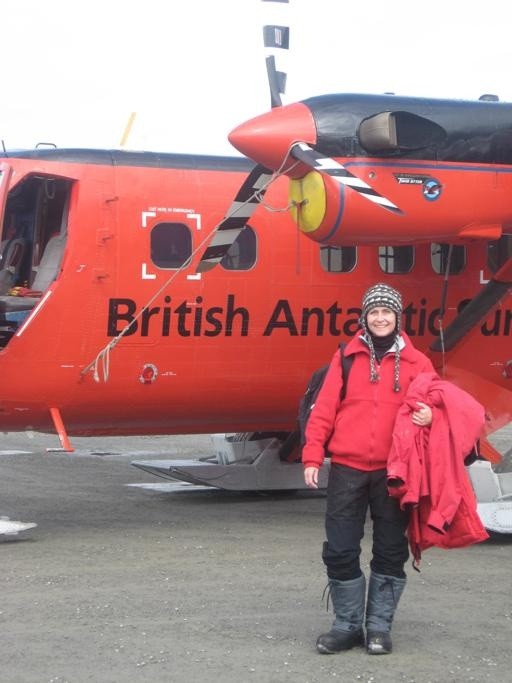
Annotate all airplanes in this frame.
[0,56,512,537]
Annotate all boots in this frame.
[364,570,408,655]
[315,571,366,655]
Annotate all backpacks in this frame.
[298,341,355,459]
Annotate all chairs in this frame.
[1,235,66,322]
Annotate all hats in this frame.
[359,281,403,336]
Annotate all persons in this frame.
[301,283,442,655]
